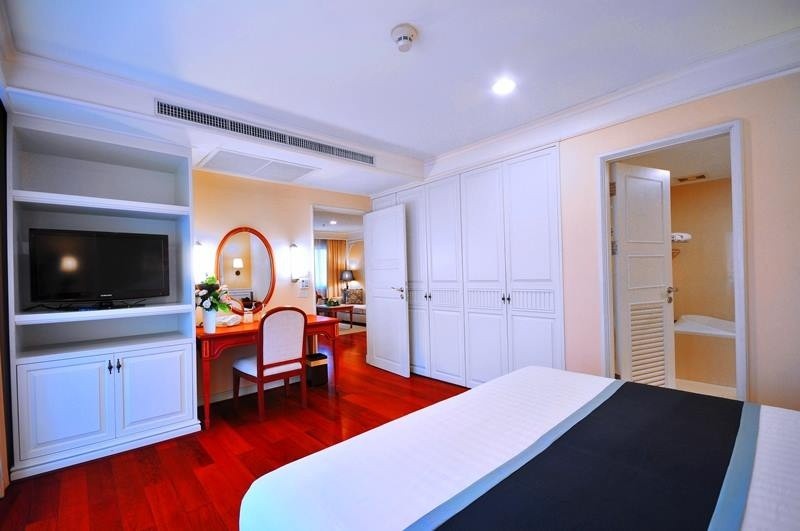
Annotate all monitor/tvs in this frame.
[28,227,170,311]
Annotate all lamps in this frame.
[340,271,355,290]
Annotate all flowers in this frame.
[194,274,232,315]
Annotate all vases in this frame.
[201,307,217,334]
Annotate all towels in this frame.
[216,314,242,326]
[672,233,692,241]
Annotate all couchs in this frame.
[337,297,366,324]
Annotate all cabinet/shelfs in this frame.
[10,343,201,483]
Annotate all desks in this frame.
[196,314,340,430]
[317,305,354,329]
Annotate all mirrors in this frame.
[215,227,276,313]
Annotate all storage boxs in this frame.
[345,289,363,304]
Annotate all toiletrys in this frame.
[258,303,269,322]
[251,302,257,309]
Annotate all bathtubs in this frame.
[674,315,736,388]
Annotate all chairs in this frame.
[232,306,308,420]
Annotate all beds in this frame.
[240,364,800,531]
